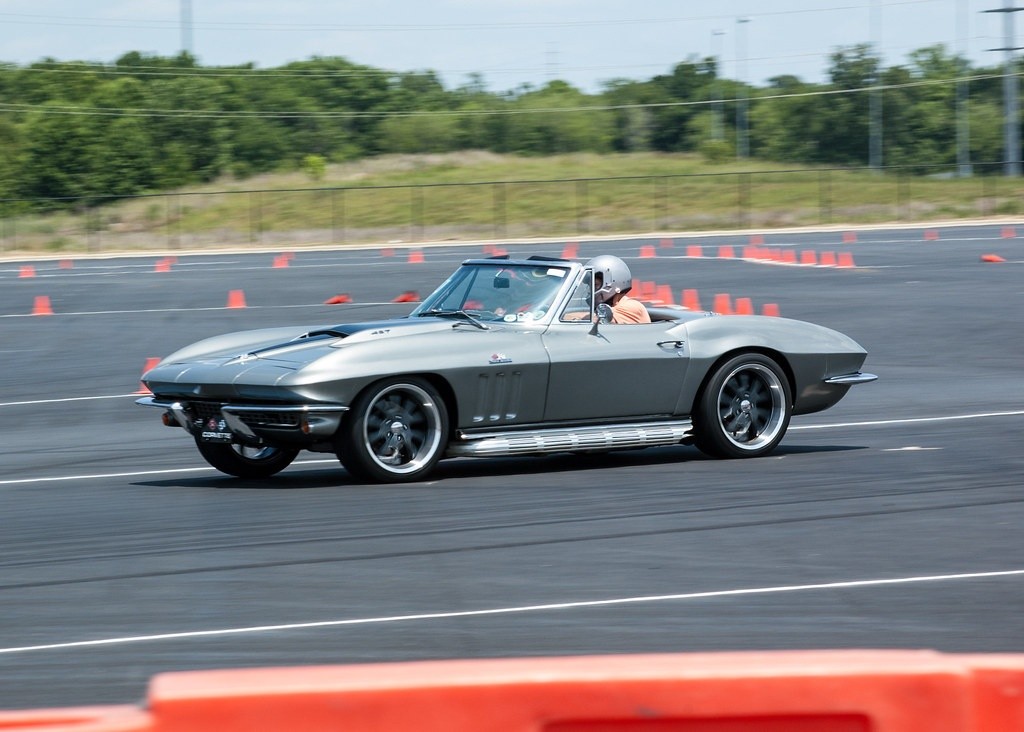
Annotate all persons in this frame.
[575,254,653,323]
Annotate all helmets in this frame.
[580,255,633,311]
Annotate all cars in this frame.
[133,256,880,486]
